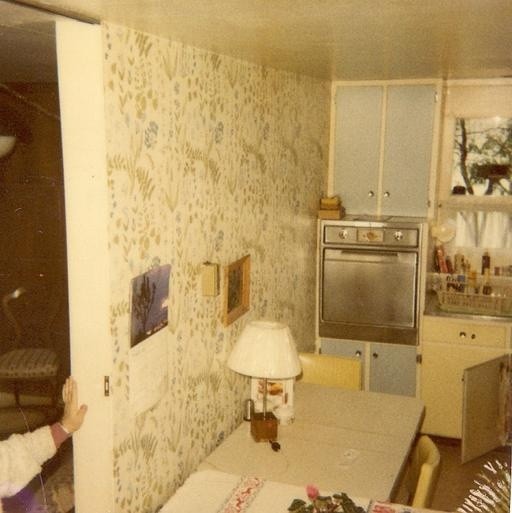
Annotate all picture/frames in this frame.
[250,376,293,410]
[223,254,250,326]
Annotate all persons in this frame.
[0,377,88,496]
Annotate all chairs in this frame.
[1,278,66,407]
[392,435,441,510]
[296,352,361,392]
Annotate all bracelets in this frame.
[57,419,72,437]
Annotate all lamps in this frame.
[226,320,303,443]
[0,104,33,157]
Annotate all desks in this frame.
[155,380,426,513]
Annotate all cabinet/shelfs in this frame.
[418,307,507,439]
[315,337,417,399]
[327,78,444,219]
